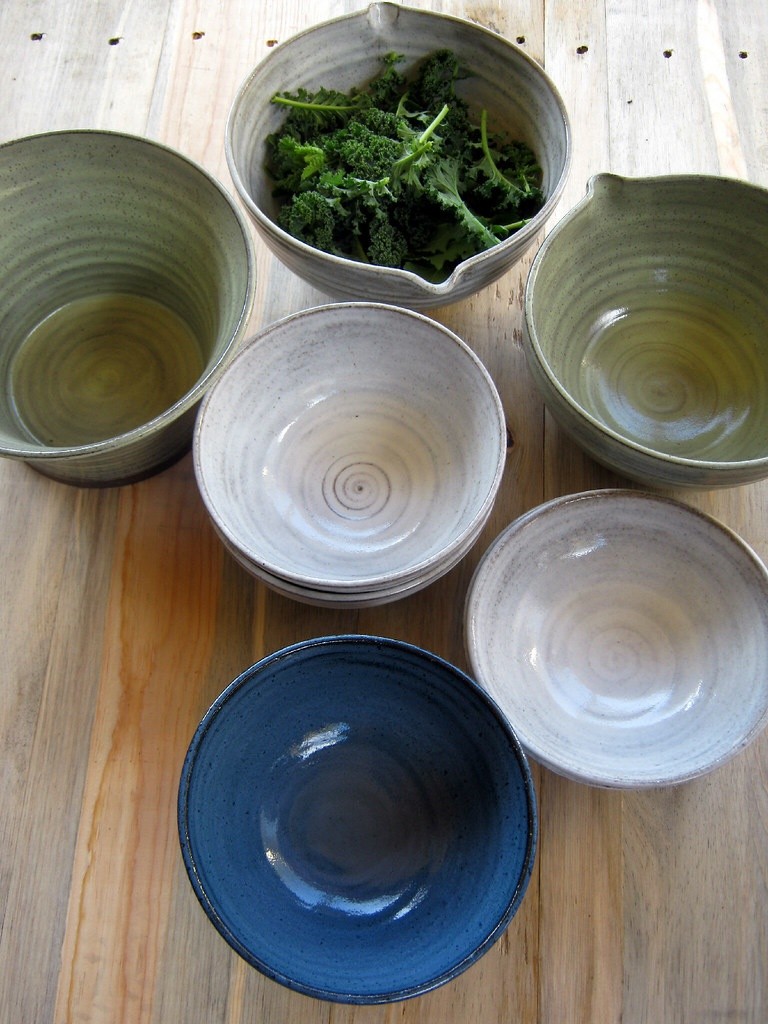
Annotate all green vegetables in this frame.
[267,50,545,273]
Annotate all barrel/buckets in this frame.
[0,126,256,488]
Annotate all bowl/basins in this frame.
[224,1,574,311]
[192,299,507,593]
[463,488,768,792]
[520,170,768,491]
[176,634,539,1006]
